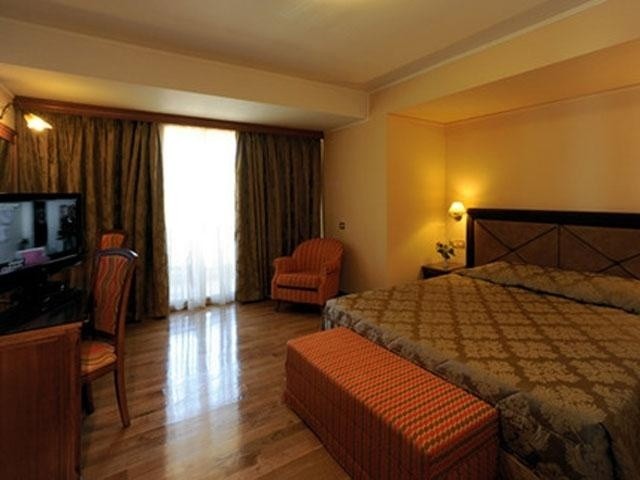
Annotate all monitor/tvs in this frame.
[0,191,87,304]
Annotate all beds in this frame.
[317,209,639,471]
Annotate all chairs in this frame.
[78,226,140,429]
[272,237,345,316]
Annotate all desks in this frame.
[1,286,92,479]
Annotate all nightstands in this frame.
[422,260,467,278]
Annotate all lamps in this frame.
[0,93,54,133]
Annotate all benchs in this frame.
[279,324,500,475]
